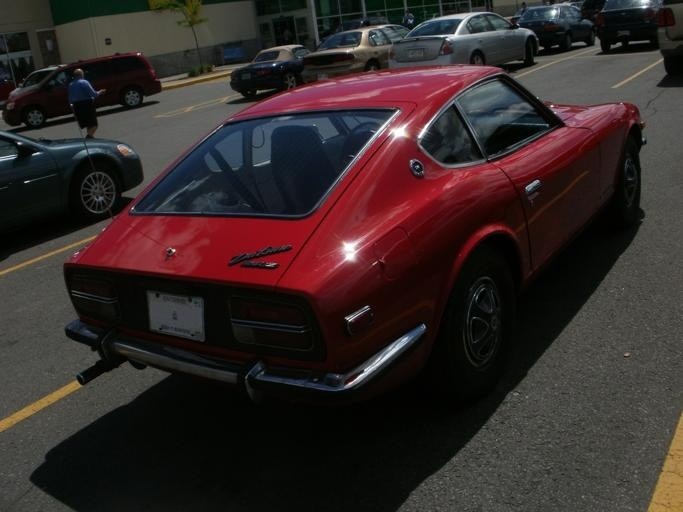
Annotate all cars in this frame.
[0,130,143,227]
[517,7,596,51]
[656,0,683,75]
[596,0,663,49]
[388,12,538,68]
[231,45,312,95]
[302,23,409,83]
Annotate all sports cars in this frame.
[64,64,646,408]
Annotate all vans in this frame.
[3,53,161,126]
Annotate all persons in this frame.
[404,9,415,30]
[282,27,292,46]
[516,2,528,14]
[68,68,109,140]
